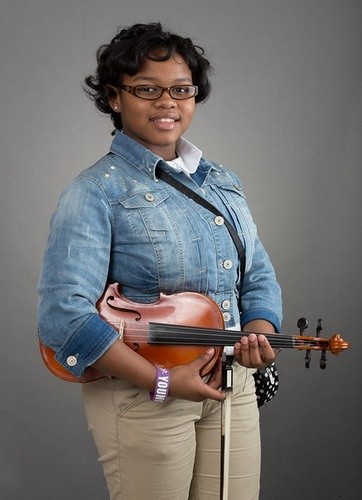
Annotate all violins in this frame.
[39,283,349,382]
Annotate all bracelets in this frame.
[149,367,170,403]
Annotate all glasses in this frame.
[119,84,199,101]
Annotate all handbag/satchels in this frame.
[254,361,279,407]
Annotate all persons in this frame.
[36,21,284,500]
